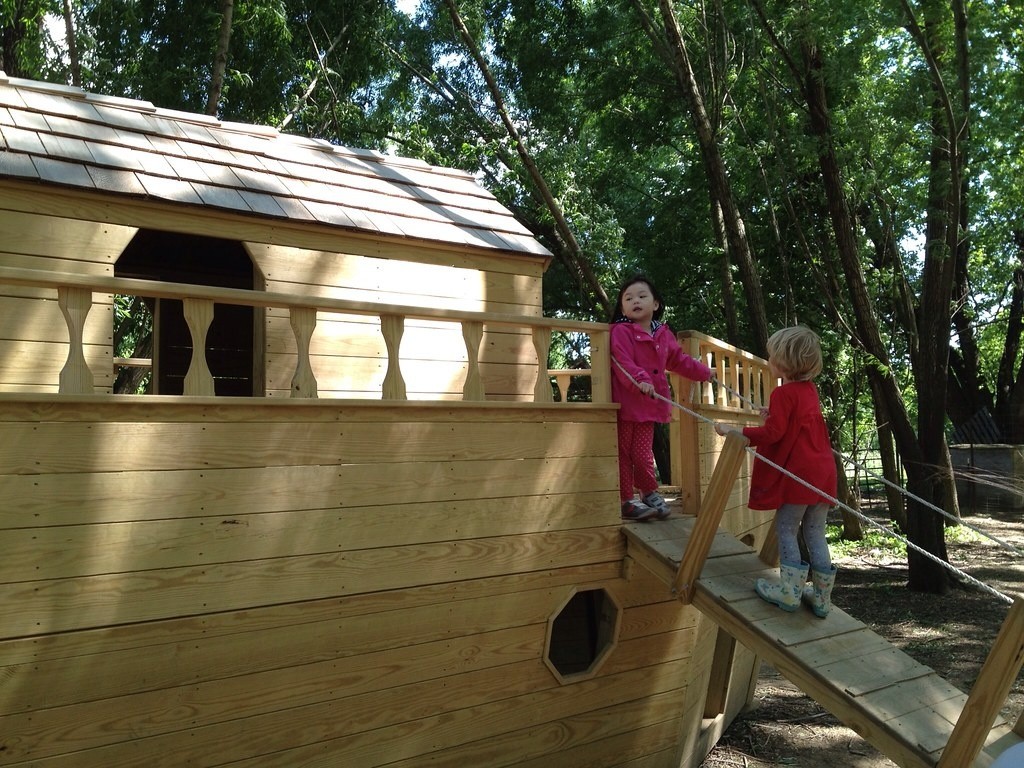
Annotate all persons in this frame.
[610,277,716,521]
[714,326,838,618]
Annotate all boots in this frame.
[803,566,837,618]
[755,560,810,612]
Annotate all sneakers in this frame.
[621,492,671,519]
